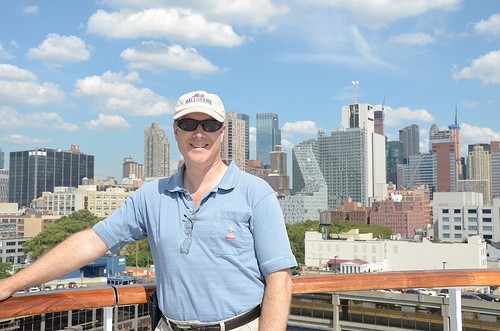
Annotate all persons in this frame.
[0,90,298,331]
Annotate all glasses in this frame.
[174,119,224,132]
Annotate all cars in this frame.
[55,284,65,290]
[45,286,51,291]
[18,289,26,294]
[461,293,500,302]
[67,282,78,289]
[372,288,449,299]
[30,287,39,293]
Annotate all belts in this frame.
[161,305,261,331]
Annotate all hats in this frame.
[174,91,225,122]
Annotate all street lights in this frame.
[334,256,339,274]
[443,262,447,269]
